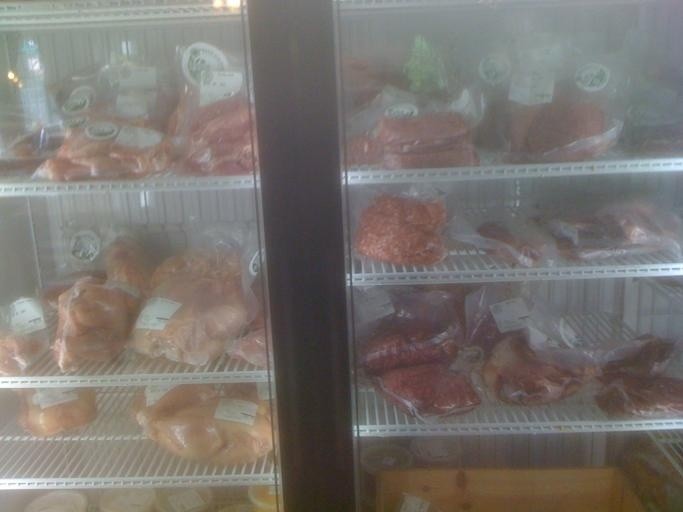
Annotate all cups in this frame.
[23,485,284,512]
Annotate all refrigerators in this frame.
[0,0,682,512]
